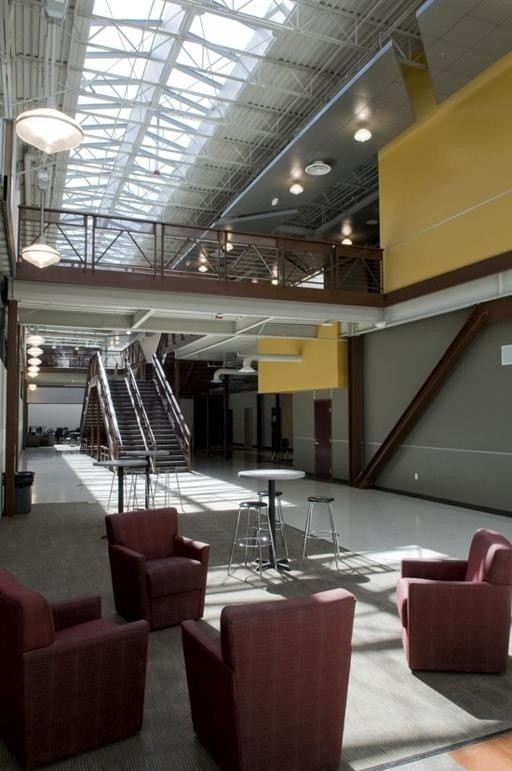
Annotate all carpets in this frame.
[0,502,511,769]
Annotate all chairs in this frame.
[180,588,356,771]
[1,566,148,771]
[105,507,209,630]
[396,529,512,675]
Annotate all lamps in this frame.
[341,236,352,246]
[354,126,371,144]
[10,103,85,157]
[289,181,303,194]
[223,242,234,254]
[20,171,61,269]
[198,263,208,273]
[25,330,46,392]
[306,160,329,178]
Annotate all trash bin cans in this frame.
[3,471,34,514]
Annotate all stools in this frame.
[254,490,289,556]
[301,495,341,570]
[95,447,185,510]
[227,501,278,580]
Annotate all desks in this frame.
[238,470,306,570]
